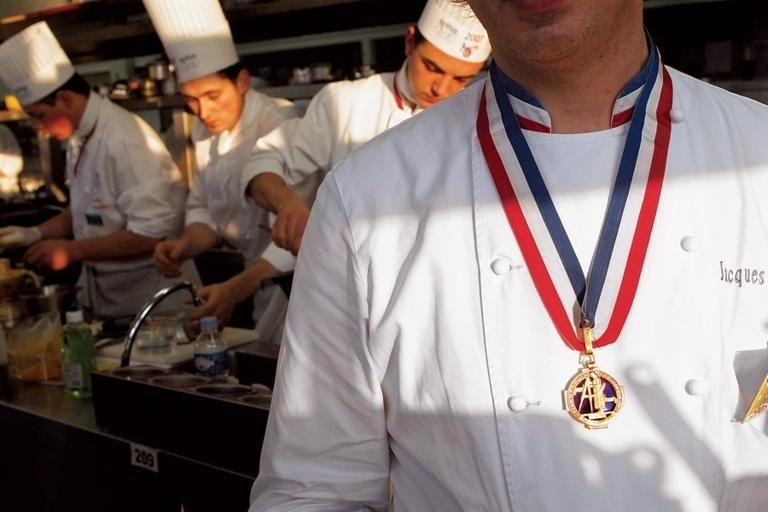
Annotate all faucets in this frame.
[118,279,201,367]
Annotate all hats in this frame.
[1,21,76,107]
[141,0,240,83]
[417,0,492,63]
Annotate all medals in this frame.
[561,326,622,432]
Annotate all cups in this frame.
[132,317,179,356]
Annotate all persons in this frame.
[235,25,486,356]
[152,60,312,349]
[245,0,768,511]
[0,74,209,318]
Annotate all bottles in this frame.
[193,316,229,376]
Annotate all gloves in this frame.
[0,226,44,254]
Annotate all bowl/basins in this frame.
[17,284,85,314]
